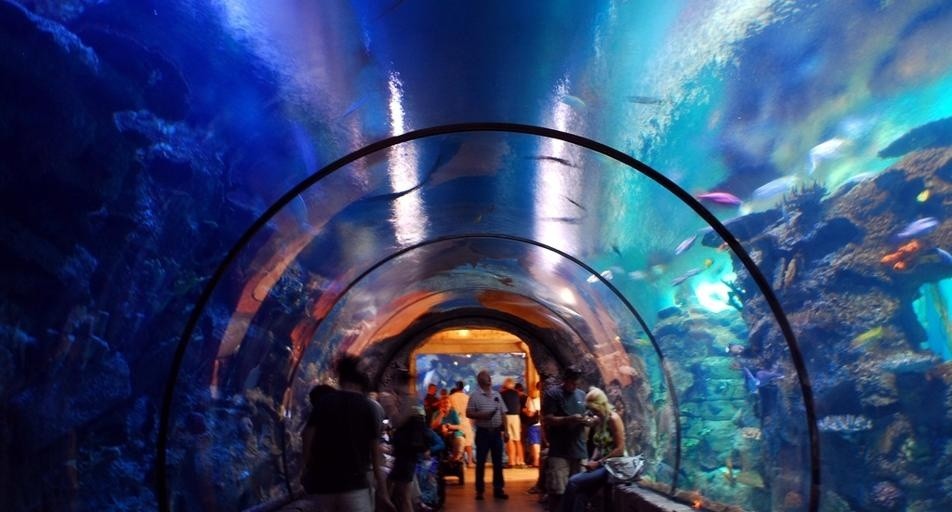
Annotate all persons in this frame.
[424,360,448,388]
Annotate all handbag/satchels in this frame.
[603,452,646,484]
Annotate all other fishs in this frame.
[587,230,741,287]
[697,190,746,208]
[882,176,950,274]
[851,324,886,349]
